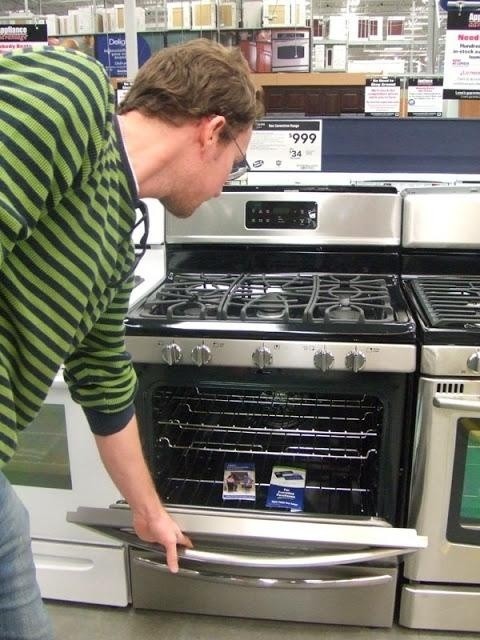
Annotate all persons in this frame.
[242,472,253,493]
[226,473,237,491]
[0,36,267,640]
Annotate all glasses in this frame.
[224,128,250,181]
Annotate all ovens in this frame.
[66,335,480,589]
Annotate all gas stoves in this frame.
[126,268,480,342]
